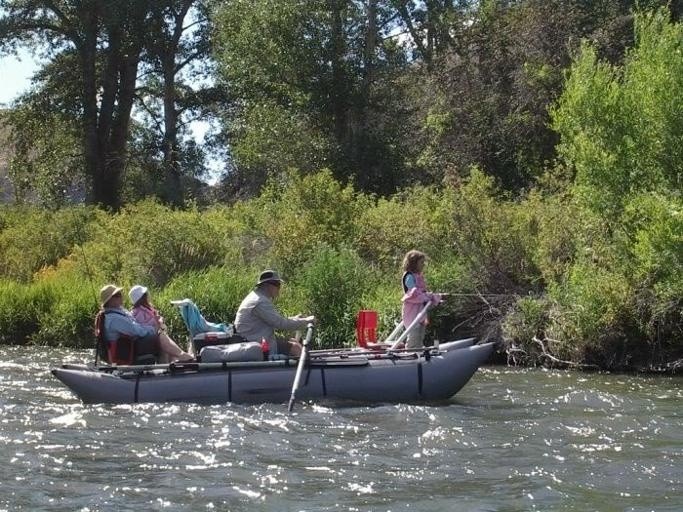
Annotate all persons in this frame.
[400,249,441,352]
[126,285,169,364]
[232,269,314,361]
[94,284,193,366]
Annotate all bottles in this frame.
[260,335,270,362]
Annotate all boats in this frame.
[52,337,496,406]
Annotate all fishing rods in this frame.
[69,201,101,314]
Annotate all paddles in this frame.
[169,357,369,368]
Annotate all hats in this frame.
[100,285,123,307]
[129,285,147,305]
[255,271,283,287]
